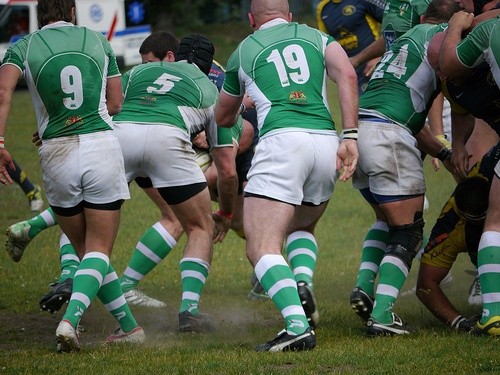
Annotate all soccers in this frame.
[192,149,212,173]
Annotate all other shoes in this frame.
[435,134,452,151]
[248,281,268,301]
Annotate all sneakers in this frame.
[350,286,375,319]
[459,319,475,334]
[253,328,316,353]
[55,319,80,354]
[297,281,319,328]
[105,326,145,343]
[468,274,483,305]
[470,315,500,338]
[178,311,214,332]
[124,289,167,308]
[40,278,73,315]
[5,221,32,263]
[366,311,411,337]
[26,184,44,218]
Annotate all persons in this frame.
[0,0,270,350]
[214,0,359,351]
[317,0,500,335]
